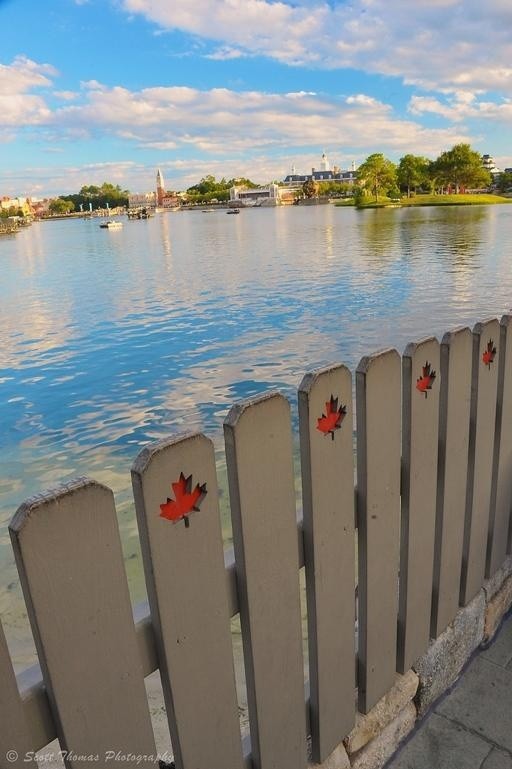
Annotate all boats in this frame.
[201,207,216,212]
[100,220,122,228]
[226,209,240,214]
[126,207,154,219]
